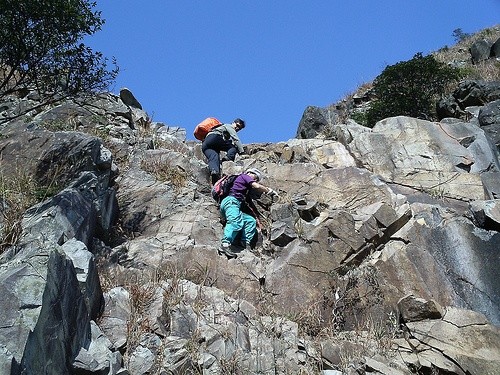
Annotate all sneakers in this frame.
[241,241,252,251]
[218,243,237,259]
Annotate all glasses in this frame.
[236,123,241,131]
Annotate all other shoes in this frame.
[211,171,220,186]
[222,156,231,161]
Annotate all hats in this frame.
[246,168,261,182]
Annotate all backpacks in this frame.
[193,117,224,141]
[211,174,239,210]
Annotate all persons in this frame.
[202,118,245,186]
[219,167,279,258]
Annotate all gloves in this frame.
[263,187,278,198]
